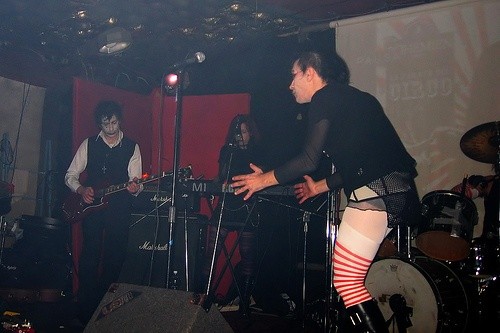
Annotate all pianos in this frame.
[161,177,296,197]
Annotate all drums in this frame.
[376,220,407,257]
[469,246,500,278]
[364,256,468,333]
[415,189,477,262]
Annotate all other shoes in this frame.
[70,308,83,327]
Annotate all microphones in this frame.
[172,52,205,69]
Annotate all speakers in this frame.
[83,283,234,333]
[118,214,209,293]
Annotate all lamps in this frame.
[95,27,135,56]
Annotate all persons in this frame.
[202,46,500,333]
[64,101,144,331]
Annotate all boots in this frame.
[238,274,255,321]
[348,300,389,333]
[199,265,211,293]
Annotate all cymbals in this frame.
[459,121,500,164]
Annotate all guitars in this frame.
[60,167,191,224]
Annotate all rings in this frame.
[134,188,137,191]
